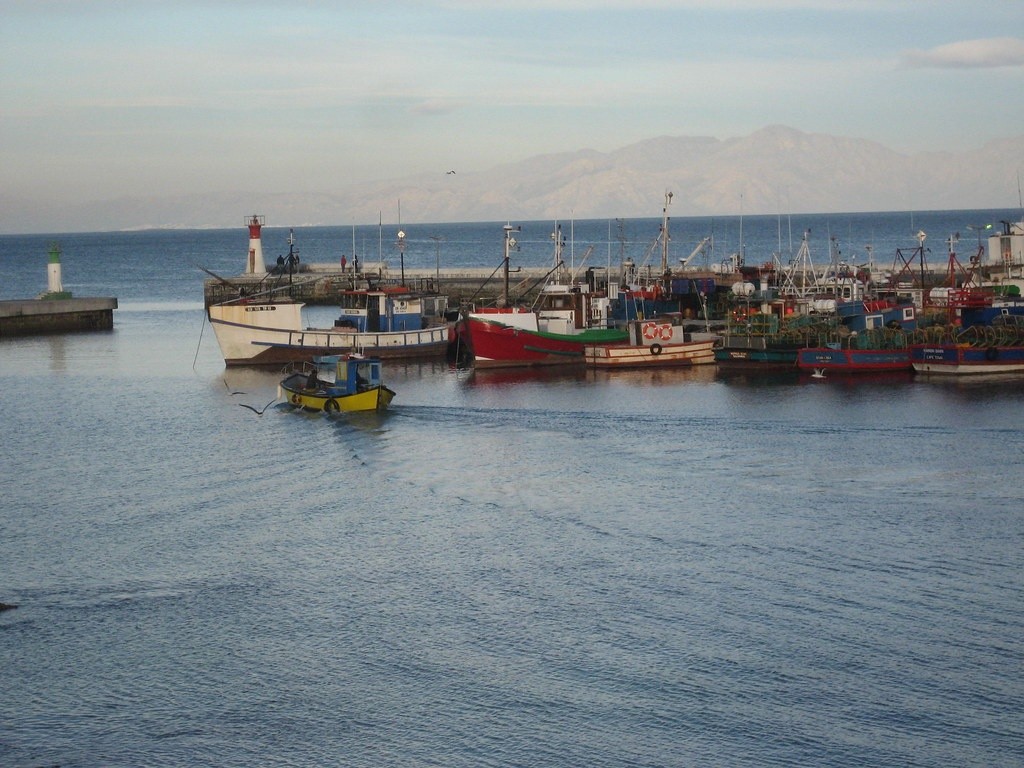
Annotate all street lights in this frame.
[916,231,927,288]
[398,229,406,286]
[964,224,994,293]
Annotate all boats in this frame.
[278,346,398,416]
[206,187,1024,386]
